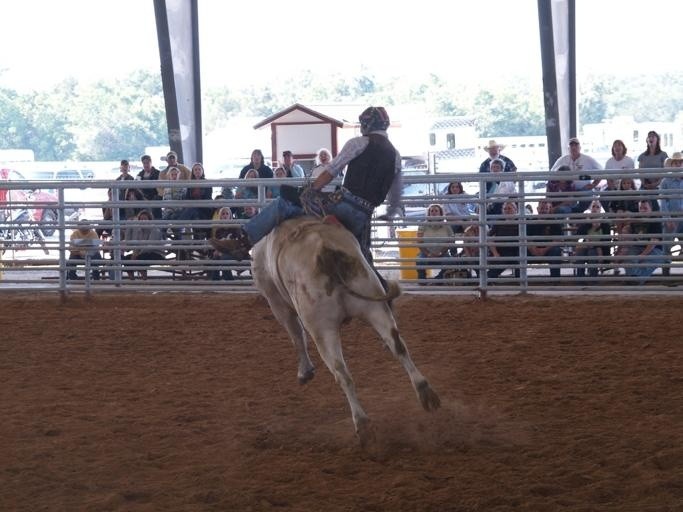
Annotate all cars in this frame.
[0,169,59,236]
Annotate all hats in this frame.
[483,140,506,153]
[569,138,579,145]
[664,152,683,167]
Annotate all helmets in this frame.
[359,107,390,136]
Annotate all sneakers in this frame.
[209,238,249,262]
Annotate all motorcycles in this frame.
[0,209,49,256]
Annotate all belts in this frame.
[340,186,375,212]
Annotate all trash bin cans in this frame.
[397,230,431,278]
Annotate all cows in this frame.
[248,211,442,449]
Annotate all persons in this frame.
[460,130,683,286]
[416,181,470,285]
[67,148,344,281]
[208,106,407,310]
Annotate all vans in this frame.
[34,169,99,202]
